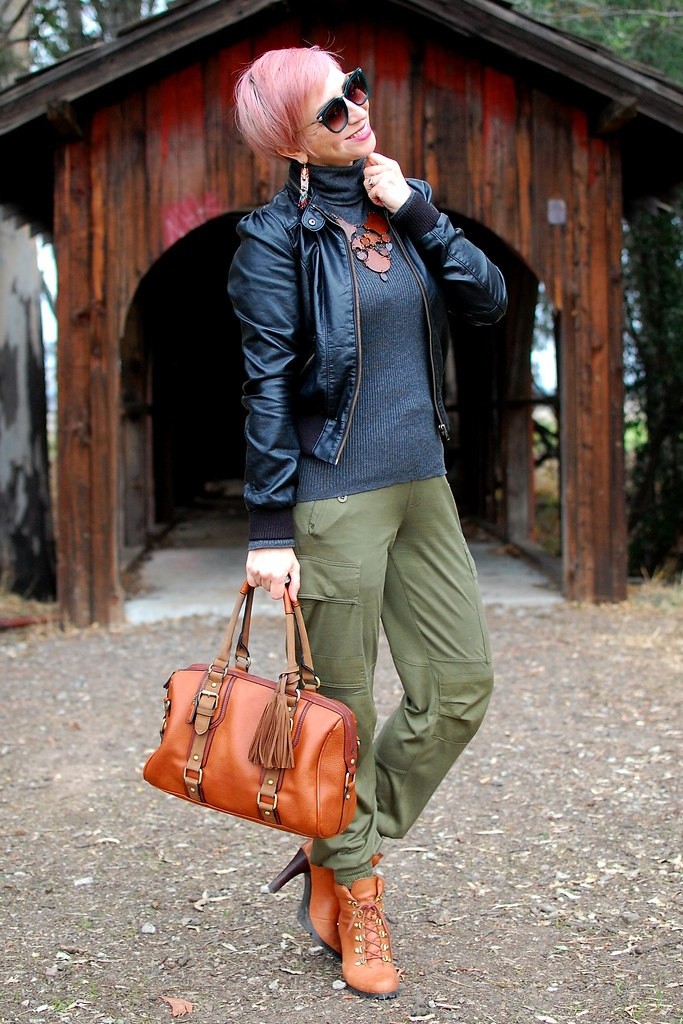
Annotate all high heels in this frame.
[268,839,383,959]
[334,873,399,1000]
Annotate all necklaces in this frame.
[329,211,393,282]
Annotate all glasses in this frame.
[295,67,372,134]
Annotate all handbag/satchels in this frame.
[143,578,358,839]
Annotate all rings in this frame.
[368,177,375,188]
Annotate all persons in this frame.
[227,46,509,1000]
